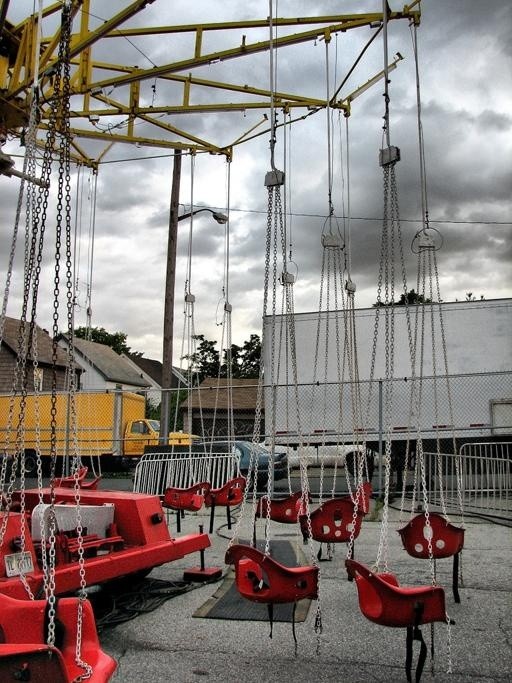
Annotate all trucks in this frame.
[0,388,200,479]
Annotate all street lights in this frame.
[160,207,231,443]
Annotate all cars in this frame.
[132,439,288,492]
[250,437,386,477]
